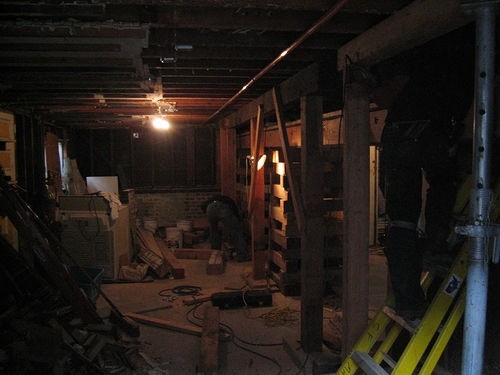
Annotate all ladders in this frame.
[335,172,499,374]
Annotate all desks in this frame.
[55,196,131,283]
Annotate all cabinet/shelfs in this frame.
[262,149,350,296]
